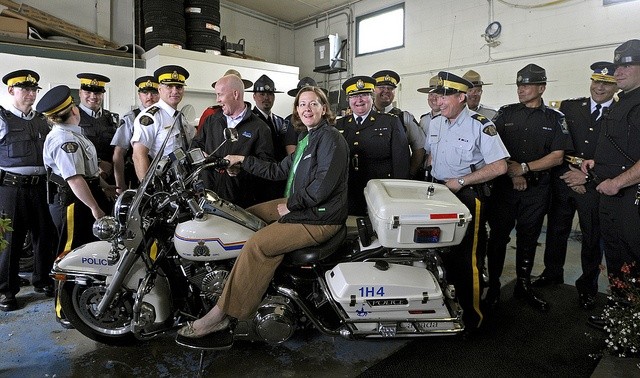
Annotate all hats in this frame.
[433,71,473,95]
[2,69,42,89]
[416,75,439,93]
[507,63,558,85]
[36,84,74,116]
[612,39,640,66]
[244,74,284,92]
[211,69,253,88]
[135,75,159,90]
[372,69,401,87]
[287,77,328,97]
[77,73,110,92]
[341,76,377,94]
[154,65,190,85]
[462,70,493,86]
[590,61,618,83]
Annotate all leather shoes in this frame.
[585,314,611,330]
[0,289,18,313]
[574,276,598,311]
[531,269,564,287]
[513,279,550,313]
[177,318,230,338]
[483,289,501,309]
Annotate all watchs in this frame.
[520,163,530,175]
[97,157,103,167]
[458,174,468,188]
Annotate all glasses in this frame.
[20,88,40,93]
[467,87,483,96]
[349,93,371,99]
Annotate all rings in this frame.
[567,179,571,184]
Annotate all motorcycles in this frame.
[49,102,472,351]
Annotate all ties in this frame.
[356,116,362,126]
[267,116,274,126]
[591,104,601,122]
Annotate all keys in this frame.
[634,188,640,206]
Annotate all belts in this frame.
[564,154,585,170]
[3,172,47,189]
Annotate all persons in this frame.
[583,39,640,329]
[190,74,274,207]
[111,77,158,191]
[36,85,121,297]
[531,62,619,307]
[77,74,119,187]
[481,63,576,310]
[335,73,410,216]
[280,76,333,159]
[426,71,512,335]
[176,86,350,351]
[372,69,426,176]
[130,64,197,183]
[1,70,52,310]
[416,75,441,131]
[462,70,498,120]
[243,74,284,132]
[197,69,252,136]
